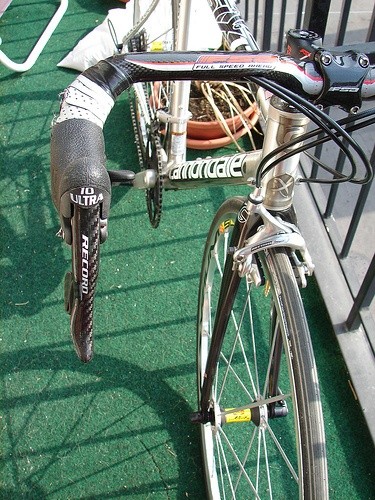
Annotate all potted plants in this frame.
[146,80,266,156]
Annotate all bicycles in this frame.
[50,0,375,500]
